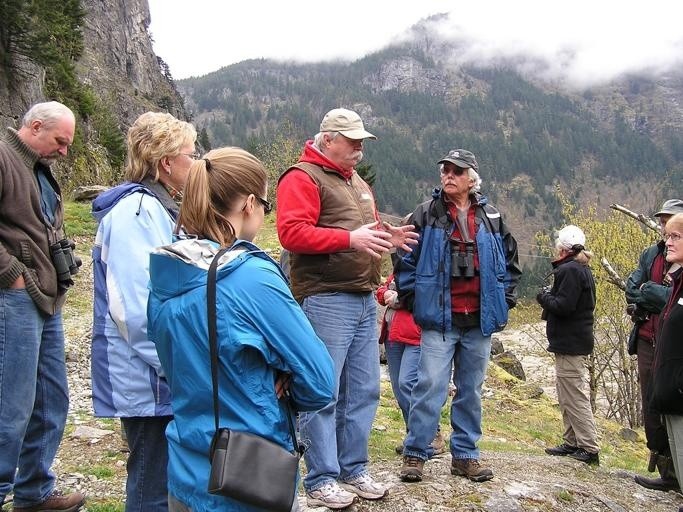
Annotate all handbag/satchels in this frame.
[208,428,300,512]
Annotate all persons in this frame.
[626,199,683,492]
[277,248,305,457]
[146,147,336,511]
[91,113,200,510]
[536,224,601,465]
[648,212,683,511]
[274,108,421,509]
[376,212,446,457]
[0,100,87,511]
[391,148,523,481]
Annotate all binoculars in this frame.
[450,238,474,279]
[50,239,79,282]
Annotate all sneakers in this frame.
[395,431,446,455]
[338,472,389,500]
[545,443,579,456]
[400,456,426,482]
[307,482,359,509]
[451,456,494,482]
[13,489,85,512]
[566,449,599,465]
[634,474,681,492]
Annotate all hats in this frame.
[436,149,479,171]
[320,107,377,141]
[653,198,683,215]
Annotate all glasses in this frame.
[658,220,669,225]
[176,151,201,160]
[242,194,272,216]
[663,232,683,240]
[439,166,472,175]
